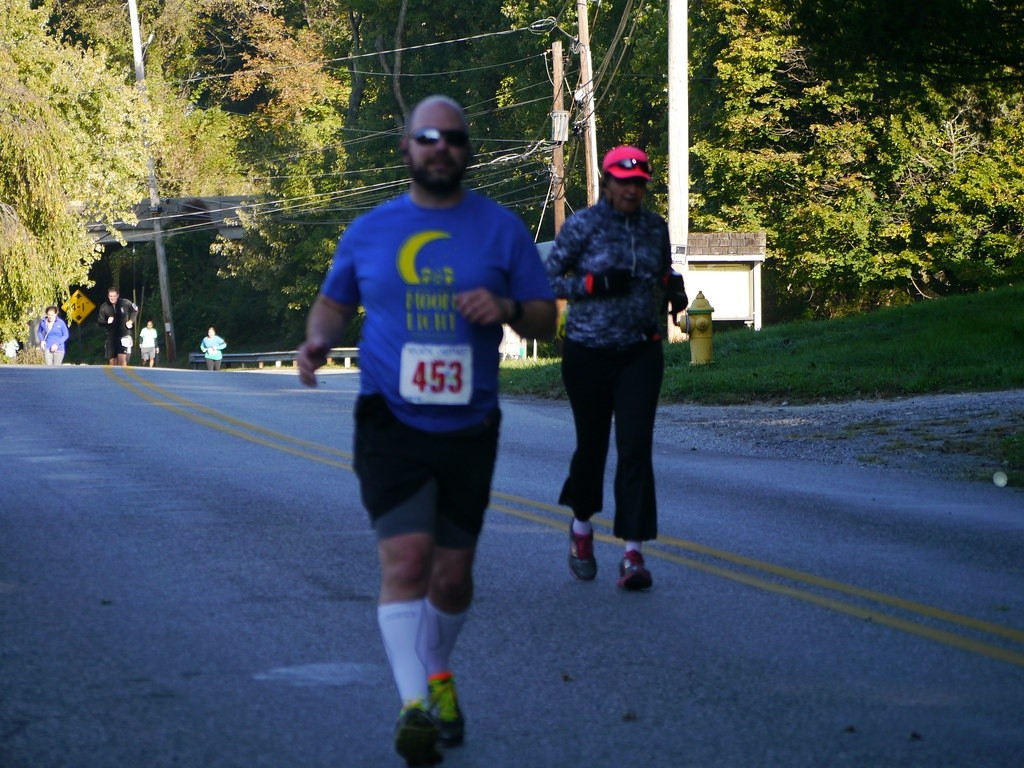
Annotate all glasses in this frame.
[411,127,469,148]
[616,159,654,174]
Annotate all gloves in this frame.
[666,274,687,311]
[584,265,635,299]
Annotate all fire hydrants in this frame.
[676,290,717,368]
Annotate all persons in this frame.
[139,321,159,368]
[545,147,688,591]
[121,335,133,366]
[295,96,559,767]
[37,307,69,365]
[200,327,227,371]
[98,288,139,366]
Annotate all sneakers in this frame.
[615,549,653,589]
[567,516,598,583]
[391,694,445,768]
[427,670,466,747]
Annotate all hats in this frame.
[602,144,654,181]
